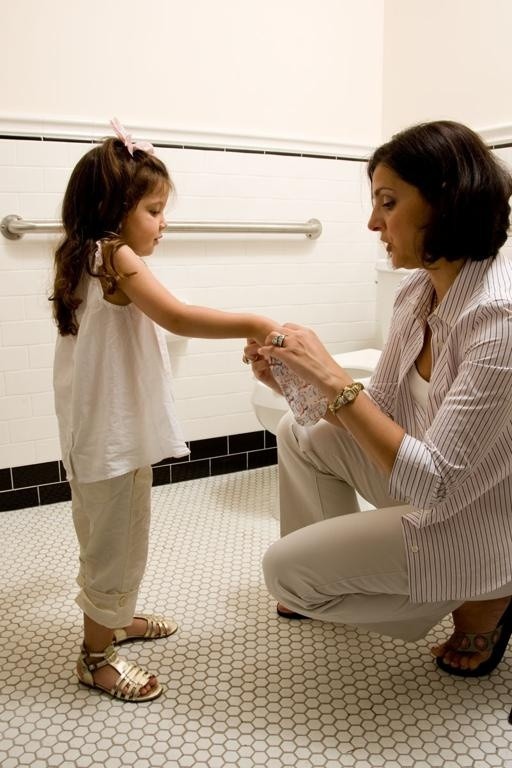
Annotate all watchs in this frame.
[328,382,364,414]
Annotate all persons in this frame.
[47,116,281,700]
[243,122,512,677]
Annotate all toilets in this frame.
[251,256,420,522]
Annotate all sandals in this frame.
[115,614,177,646]
[76,641,163,702]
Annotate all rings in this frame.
[272,335,286,347]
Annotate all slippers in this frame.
[436,599,512,678]
